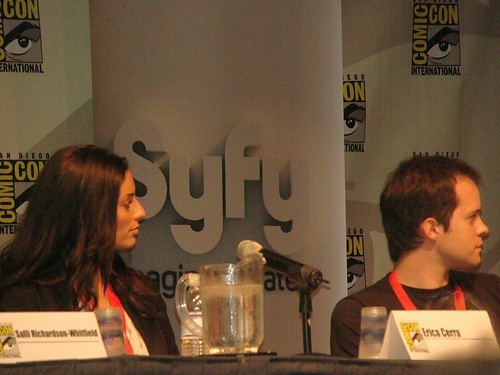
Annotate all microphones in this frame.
[236,239,330,290]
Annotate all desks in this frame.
[0,354,500,375]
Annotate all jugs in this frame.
[174,254,264,355]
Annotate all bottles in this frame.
[181,273,204,354]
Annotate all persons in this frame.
[0,144,179,357]
[330,156,500,359]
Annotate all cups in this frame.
[95,307,125,356]
[358,307,387,358]
